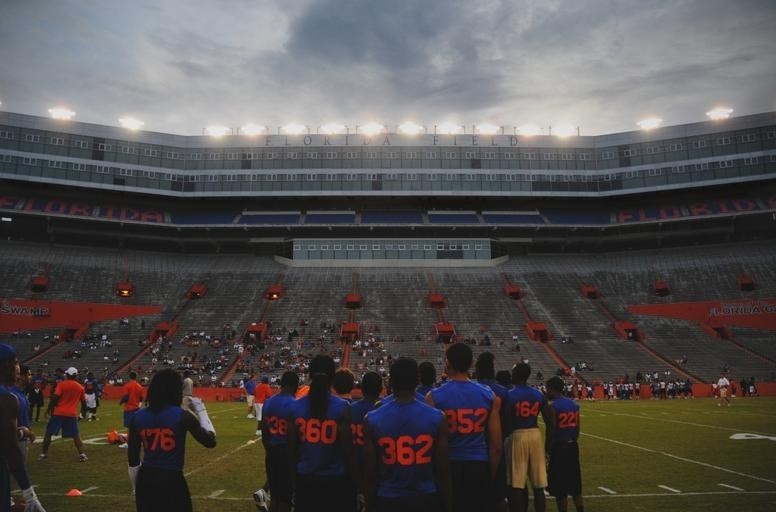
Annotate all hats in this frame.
[64,367,78,375]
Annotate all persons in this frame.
[474,354,508,512]
[10,364,36,466]
[285,355,352,512]
[239,378,245,401]
[261,371,299,512]
[253,480,270,512]
[127,368,218,512]
[512,334,518,341]
[49,367,64,417]
[32,317,233,369]
[37,366,89,462]
[505,363,548,512]
[709,360,760,407]
[530,368,547,395]
[332,367,355,404]
[350,335,392,379]
[118,372,146,449]
[422,342,504,512]
[543,376,584,512]
[102,368,125,386]
[28,368,47,422]
[298,373,304,386]
[78,372,99,421]
[436,354,443,365]
[557,351,694,400]
[194,368,224,388]
[138,374,149,385]
[233,319,344,372]
[244,374,256,419]
[276,374,281,384]
[362,357,455,511]
[270,375,276,385]
[348,371,383,512]
[182,370,194,414]
[254,375,273,436]
[417,361,438,395]
[0,342,46,512]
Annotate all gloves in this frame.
[24,487,45,512]
[187,394,206,415]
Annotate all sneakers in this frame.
[255,429,262,437]
[246,413,255,419]
[78,454,88,462]
[253,489,270,511]
[40,452,49,459]
[118,442,129,449]
